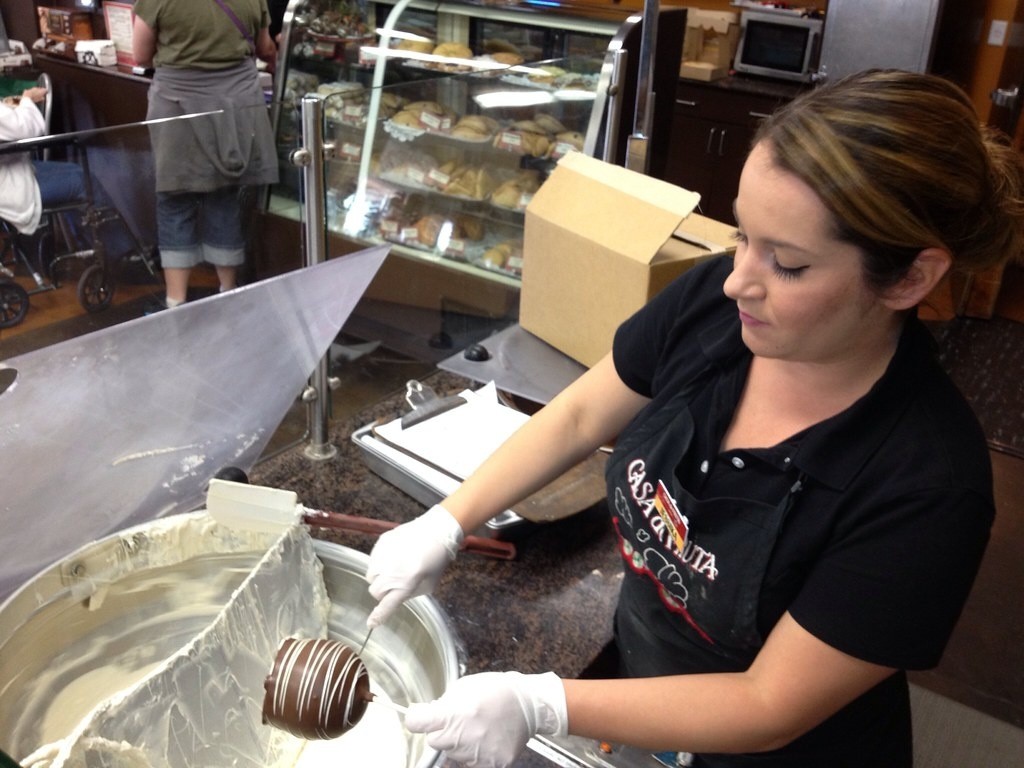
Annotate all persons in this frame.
[130,0,279,316]
[365,67,1024,768]
[0,87,131,256]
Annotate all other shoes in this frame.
[143,296,186,315]
[113,242,159,280]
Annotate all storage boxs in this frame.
[519,150,740,372]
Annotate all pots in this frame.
[0,511,460,768]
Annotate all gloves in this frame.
[366,504,464,630]
[403,669,568,768]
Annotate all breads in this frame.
[366,37,588,274]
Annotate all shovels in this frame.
[206,478,515,560]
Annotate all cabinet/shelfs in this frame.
[256,0,688,323]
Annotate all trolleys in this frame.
[1,75,128,327]
